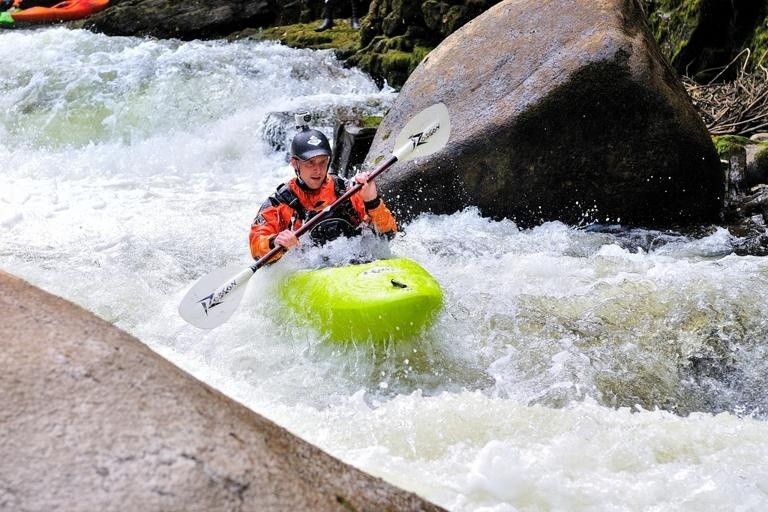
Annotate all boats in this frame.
[281,257,444,341]
[11,0,112,22]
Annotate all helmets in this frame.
[290,127,334,162]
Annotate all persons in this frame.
[247,130,399,267]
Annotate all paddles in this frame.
[178,102,450,329]
